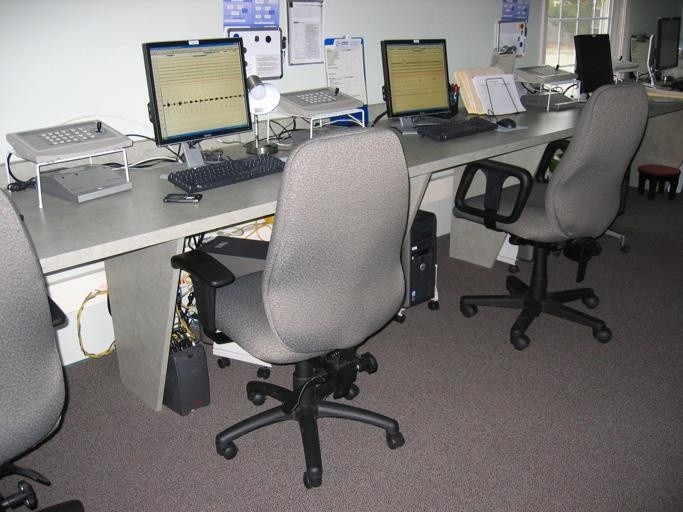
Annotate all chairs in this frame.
[171,124,413,492]
[453,80,651,353]
[0,191,83,512]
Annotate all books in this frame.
[456,66,527,115]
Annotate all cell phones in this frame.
[163,194,203,204]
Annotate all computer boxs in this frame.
[399,210,437,309]
[198,235,270,346]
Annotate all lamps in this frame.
[244,75,281,155]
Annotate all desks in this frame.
[1,88,683,410]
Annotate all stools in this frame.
[637,161,682,201]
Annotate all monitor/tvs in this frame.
[641,16,681,88]
[574,34,614,100]
[380,38,452,135]
[141,37,253,168]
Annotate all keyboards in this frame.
[168,152,293,194]
[416,116,498,143]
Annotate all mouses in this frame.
[496,118,517,130]
